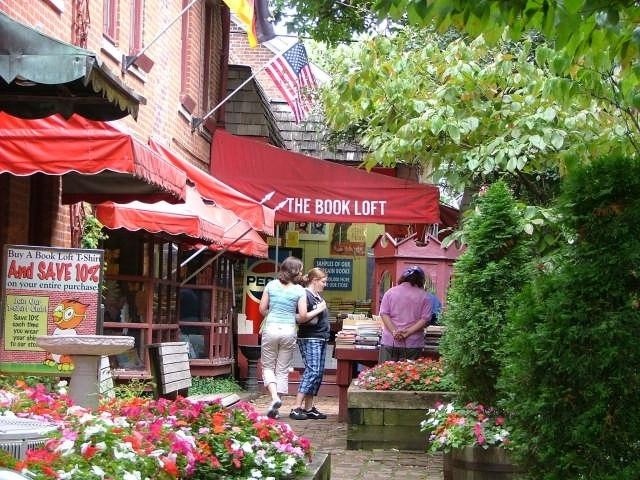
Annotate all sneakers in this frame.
[267,398,282,419]
[290,406,327,420]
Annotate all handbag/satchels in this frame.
[296,304,318,326]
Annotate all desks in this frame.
[333,346,384,419]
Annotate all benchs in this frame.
[147,339,241,417]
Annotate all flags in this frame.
[263,40,320,127]
[222,0,277,50]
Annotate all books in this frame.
[332,314,447,353]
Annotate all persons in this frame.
[178,277,204,336]
[256,255,309,421]
[423,286,442,328]
[375,265,433,367]
[289,267,333,422]
